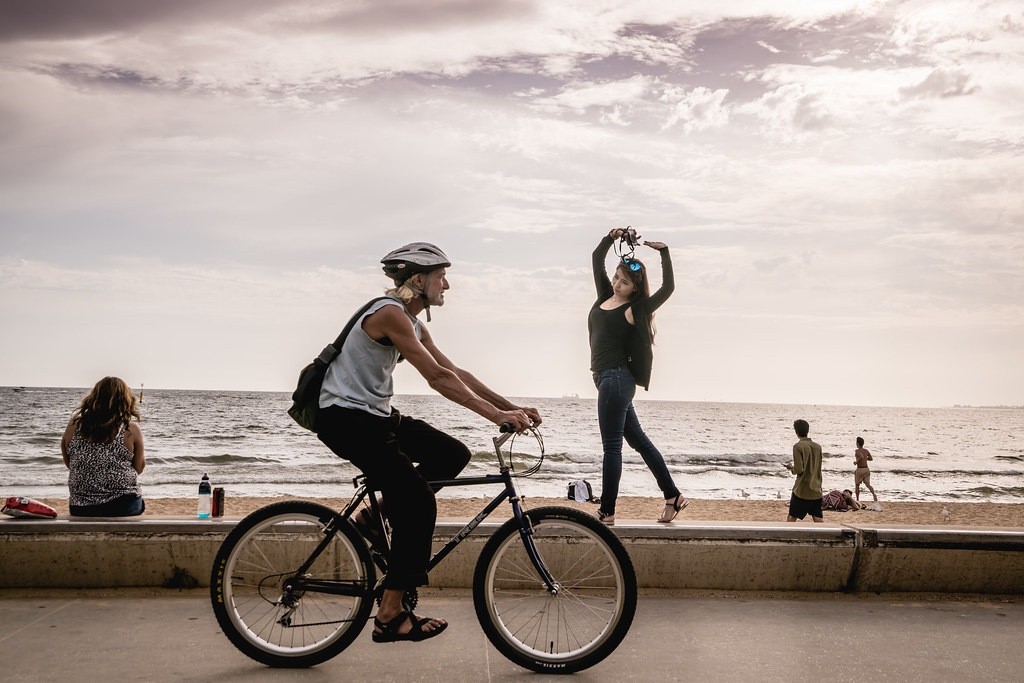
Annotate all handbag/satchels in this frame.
[288,297,398,433]
[568,480,593,503]
[0,496,57,519]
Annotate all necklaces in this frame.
[613,298,617,301]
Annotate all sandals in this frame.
[348,509,392,545]
[597,509,614,525]
[657,493,689,523]
[372,611,448,643]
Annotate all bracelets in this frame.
[609,232,618,240]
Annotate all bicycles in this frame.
[210,421,640,675]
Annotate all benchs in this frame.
[841,523,1024,595]
[0,517,337,586]
[336,518,858,590]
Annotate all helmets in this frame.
[381,242,451,299]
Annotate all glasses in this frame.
[621,260,642,274]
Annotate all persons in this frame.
[783,487,860,512]
[853,436,878,502]
[313,240,542,645]
[586,226,689,524]
[783,419,823,523]
[60,376,147,517]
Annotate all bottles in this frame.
[197,473,211,518]
